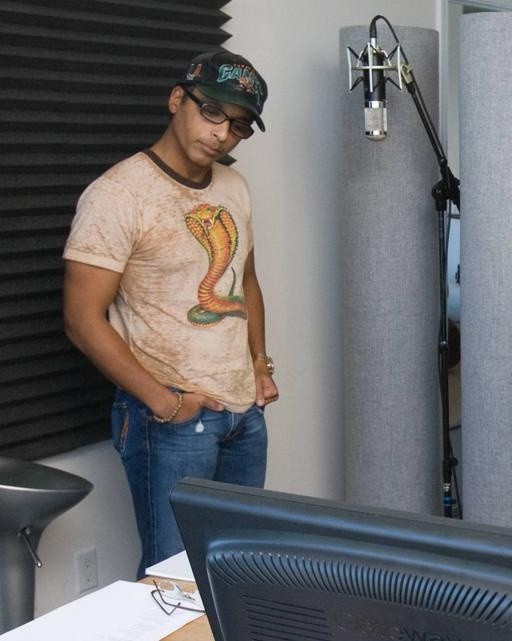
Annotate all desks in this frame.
[0,543,216,639]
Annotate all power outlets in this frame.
[75,547,98,593]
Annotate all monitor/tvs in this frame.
[168,475,512,641]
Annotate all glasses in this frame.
[181,84,254,140]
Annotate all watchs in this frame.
[251,352,274,376]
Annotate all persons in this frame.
[60,47,280,582]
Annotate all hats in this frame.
[179,50,268,134]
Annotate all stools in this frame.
[0,451,93,632]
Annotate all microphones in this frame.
[363,26,388,140]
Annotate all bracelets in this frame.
[152,391,182,424]
